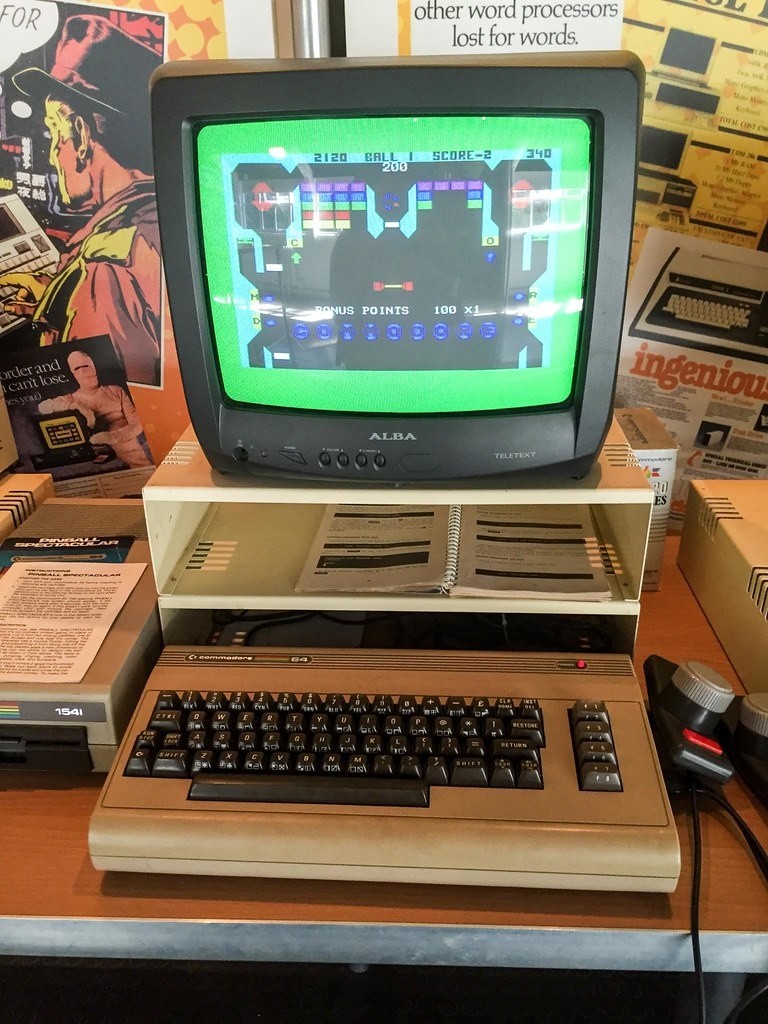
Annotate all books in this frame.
[294,505,612,602]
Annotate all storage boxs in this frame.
[613,408,680,591]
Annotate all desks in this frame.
[0,495,768,1024]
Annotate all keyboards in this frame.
[662,293,751,329]
[126,687,624,809]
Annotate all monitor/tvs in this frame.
[638,116,695,177]
[147,50,647,492]
[653,22,721,85]
